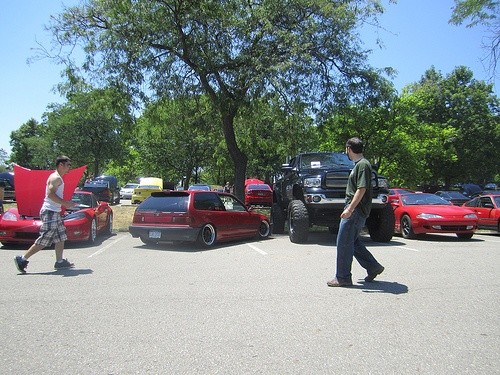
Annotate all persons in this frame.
[325,136,384,287]
[0,183,5,214]
[223,182,231,206]
[15,155,74,274]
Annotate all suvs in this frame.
[269,151,396,243]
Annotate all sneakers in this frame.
[327,274,353,287]
[14,255,29,273]
[54,258,74,269]
[365,263,385,282]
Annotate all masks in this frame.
[346,146,351,161]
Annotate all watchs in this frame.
[58,199,64,204]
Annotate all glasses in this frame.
[66,164,72,167]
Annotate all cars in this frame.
[79,175,122,205]
[128,190,271,248]
[245,184,273,209]
[0,170,17,202]
[187,184,215,193]
[388,188,417,204]
[433,191,468,207]
[462,194,500,235]
[131,177,164,204]
[119,183,140,200]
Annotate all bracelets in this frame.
[348,207,354,213]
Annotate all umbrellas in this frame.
[462,184,482,195]
[484,183,497,189]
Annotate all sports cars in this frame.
[0,163,114,247]
[389,193,479,240]
